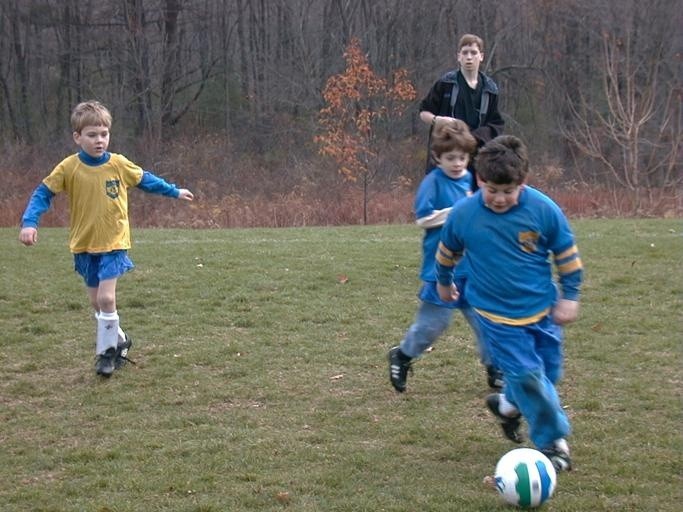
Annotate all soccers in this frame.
[492,448,557,507]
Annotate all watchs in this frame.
[431,115,437,127]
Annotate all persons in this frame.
[19,101,194,376]
[419,34,506,178]
[436,135,583,473]
[388,118,508,390]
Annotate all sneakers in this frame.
[486,394,527,445]
[94,333,135,378]
[487,365,505,389]
[388,346,413,392]
[540,448,572,472]
